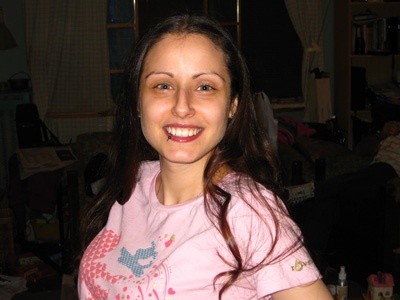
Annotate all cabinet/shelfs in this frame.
[332,0,400,152]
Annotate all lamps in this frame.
[0,6,16,51]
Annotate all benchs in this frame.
[292,135,400,203]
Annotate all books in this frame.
[352,15,400,55]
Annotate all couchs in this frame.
[68,132,317,211]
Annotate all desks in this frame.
[8,162,79,273]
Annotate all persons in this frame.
[77,15,336,300]
